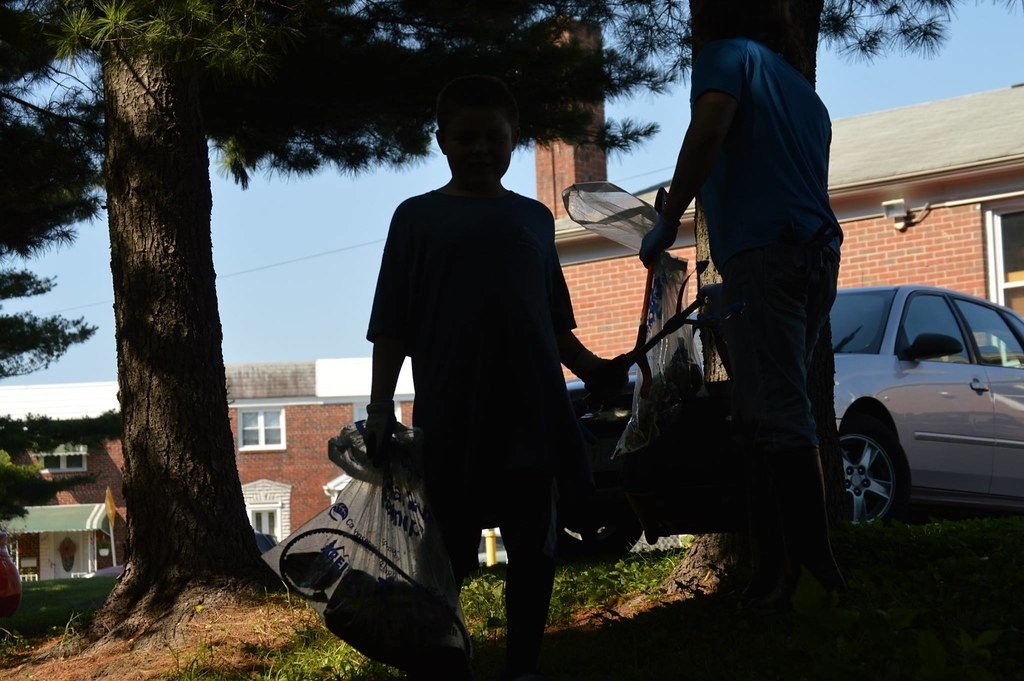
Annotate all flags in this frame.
[104,490,116,526]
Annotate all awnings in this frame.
[6,505,112,536]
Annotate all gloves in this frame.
[364,400,406,469]
[571,351,628,398]
[639,214,677,264]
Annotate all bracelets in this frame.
[367,400,395,411]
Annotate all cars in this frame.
[827,286,1023,531]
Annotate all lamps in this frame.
[882,198,909,230]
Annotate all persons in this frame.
[637,0,851,611]
[365,78,630,681]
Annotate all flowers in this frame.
[90,535,121,549]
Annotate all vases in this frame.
[99,548,109,556]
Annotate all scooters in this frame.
[555,295,754,561]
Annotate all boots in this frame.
[734,445,829,613]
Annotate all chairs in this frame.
[946,345,1002,366]
